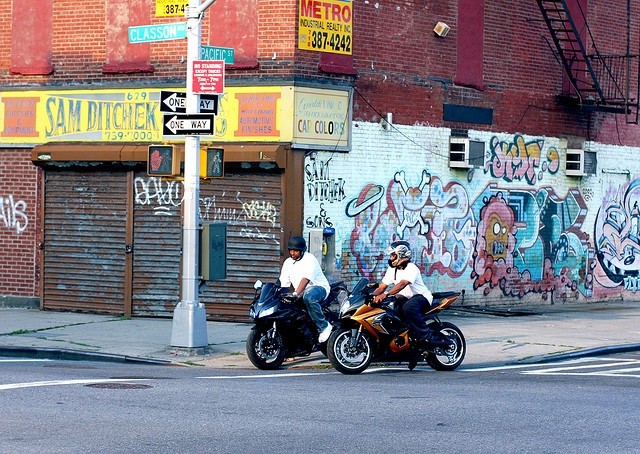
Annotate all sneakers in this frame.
[318,321,332,343]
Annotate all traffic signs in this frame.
[201,45,234,64]
[159,89,219,116]
[127,21,186,43]
[192,59,225,94]
[161,112,215,136]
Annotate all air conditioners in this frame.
[448,137,485,169]
[565,149,597,177]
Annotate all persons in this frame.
[372,240,434,344]
[277,237,334,343]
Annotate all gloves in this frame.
[285,293,297,304]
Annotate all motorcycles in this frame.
[246,279,350,370]
[326,278,466,374]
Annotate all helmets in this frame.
[287,236,306,252]
[384,241,413,268]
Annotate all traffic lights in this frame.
[199,147,224,179]
[147,145,181,176]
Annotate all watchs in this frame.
[384,291,389,296]
